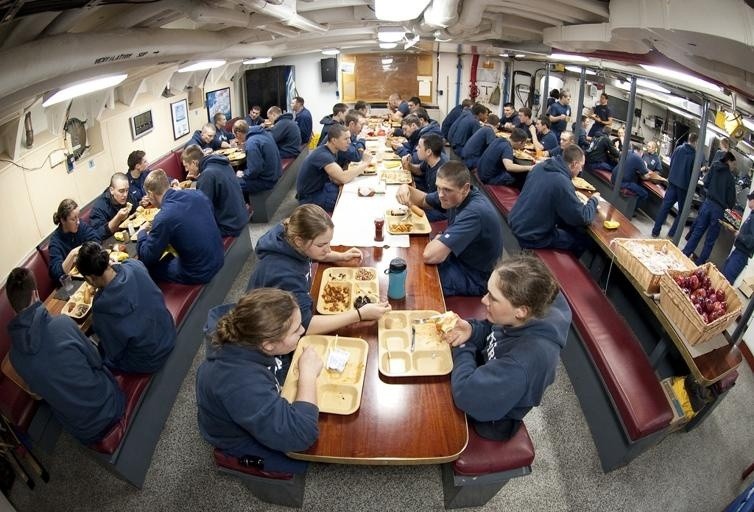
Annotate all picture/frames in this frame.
[205,87,232,125]
[169,98,190,141]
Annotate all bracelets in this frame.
[356,308,362,322]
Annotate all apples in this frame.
[674,268,730,324]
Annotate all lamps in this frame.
[242,57,272,65]
[42,74,129,107]
[322,48,340,55]
[177,59,226,75]
[498,53,526,57]
[375,0,431,22]
[379,42,397,49]
[378,28,405,43]
[547,52,596,76]
[613,62,724,95]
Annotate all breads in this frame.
[126,201,133,215]
[136,206,144,213]
[434,311,457,334]
[144,208,159,216]
[389,224,413,233]
[84,289,91,304]
[352,294,373,310]
[110,252,129,264]
[390,207,408,215]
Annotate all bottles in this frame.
[383,257,407,302]
[373,216,385,242]
[122,230,130,243]
[127,223,136,236]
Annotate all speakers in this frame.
[321,58,337,82]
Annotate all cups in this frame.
[59,274,75,295]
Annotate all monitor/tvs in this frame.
[608,95,629,123]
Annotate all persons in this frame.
[450,106,490,158]
[210,113,241,146]
[441,99,474,141]
[355,99,368,116]
[506,145,602,260]
[642,139,663,175]
[651,132,702,239]
[681,150,735,267]
[293,123,372,210]
[610,144,649,214]
[135,168,225,283]
[387,92,410,119]
[446,103,484,143]
[588,93,617,127]
[587,124,612,148]
[183,122,231,154]
[528,114,558,151]
[125,150,180,208]
[193,286,324,474]
[179,143,248,238]
[476,127,536,186]
[611,124,626,158]
[6,266,127,450]
[88,172,136,239]
[394,160,503,296]
[719,190,754,287]
[498,103,520,128]
[385,107,445,139]
[460,114,501,171]
[572,114,595,150]
[710,137,730,166]
[317,102,350,147]
[390,114,447,193]
[407,97,421,111]
[266,105,303,160]
[47,198,102,279]
[540,130,585,178]
[503,108,535,133]
[230,119,284,221]
[401,133,450,221]
[433,251,572,442]
[243,104,266,129]
[74,240,174,374]
[337,110,368,171]
[544,89,572,145]
[547,89,560,108]
[289,97,311,145]
[244,203,392,336]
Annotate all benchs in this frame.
[684,370,739,435]
[214,210,333,508]
[145,151,182,183]
[71,201,257,490]
[0,247,55,486]
[171,143,186,180]
[431,221,535,509]
[247,145,310,224]
[579,168,640,223]
[471,163,675,474]
[36,201,94,266]
